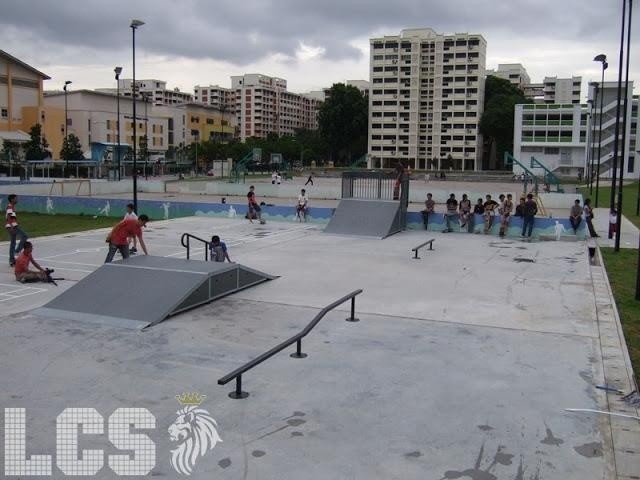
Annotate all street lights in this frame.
[291,139,304,163]
[129,18,147,215]
[113,66,123,180]
[63,80,73,178]
[193,130,199,173]
[587,53,608,208]
[220,104,225,144]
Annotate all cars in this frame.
[208,169,213,176]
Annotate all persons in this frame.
[5,196,27,267]
[248,186,262,223]
[15,241,49,282]
[208,236,235,265]
[609,210,618,238]
[422,171,600,240]
[105,215,150,263]
[297,188,309,222]
[271,169,281,185]
[305,172,314,185]
[124,203,137,254]
